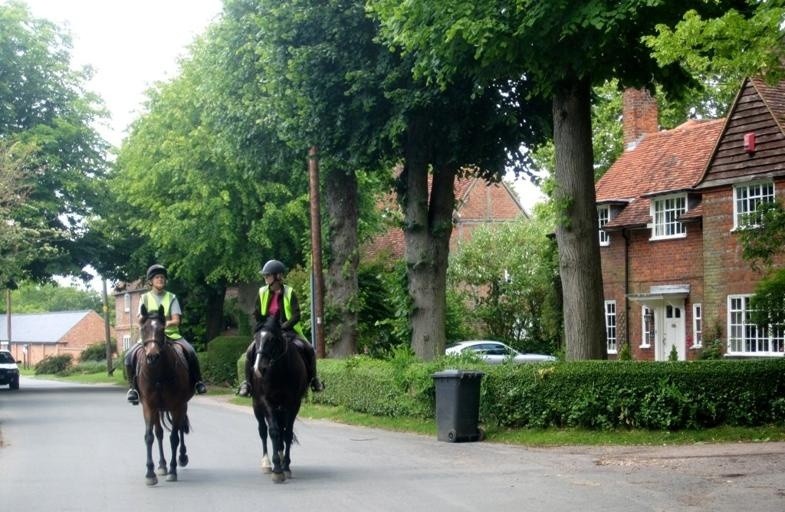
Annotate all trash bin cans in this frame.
[430,370,485,442]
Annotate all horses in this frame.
[245,308,309,484]
[132,303,195,485]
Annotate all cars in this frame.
[445,337,559,367]
[0,349,21,390]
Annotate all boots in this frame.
[307,356,323,391]
[189,358,206,394]
[241,360,254,395]
[125,365,139,402]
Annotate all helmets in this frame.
[258,260,285,275]
[148,264,168,279]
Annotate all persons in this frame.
[125,264,207,406]
[237,260,324,396]
